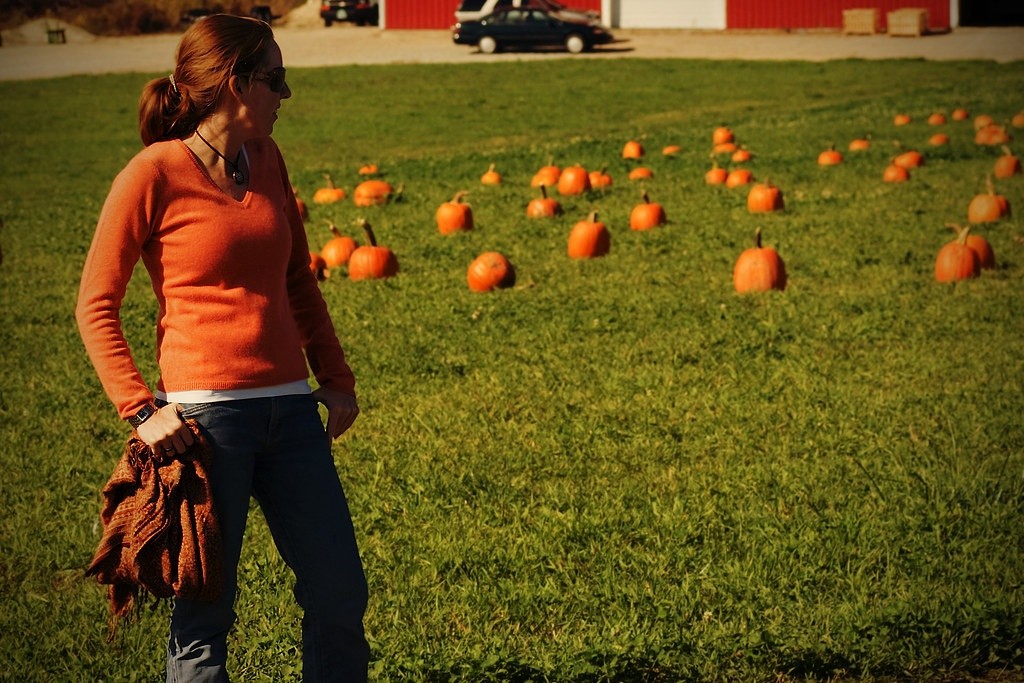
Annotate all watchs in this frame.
[127,397,161,429]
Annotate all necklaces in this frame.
[195,128,245,185]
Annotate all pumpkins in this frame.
[291,163,516,293]
[525,123,787,297]
[818,108,1024,282]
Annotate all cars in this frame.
[452,7,612,55]
[320,0,378,27]
[452,0,602,30]
[178,3,282,31]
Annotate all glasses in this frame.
[244,66,286,92]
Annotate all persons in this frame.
[73,13,373,683]
